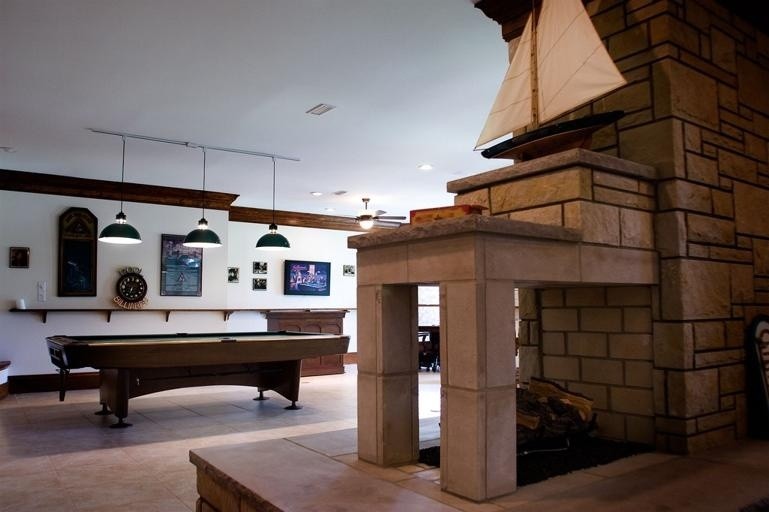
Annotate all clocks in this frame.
[114,266,150,310]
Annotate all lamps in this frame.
[98,127,290,251]
[360,219,373,231]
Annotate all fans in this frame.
[353,210,406,224]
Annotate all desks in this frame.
[44,331,351,430]
[265,311,347,376]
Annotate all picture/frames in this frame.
[283,259,332,295]
[10,247,30,269]
[159,234,203,297]
